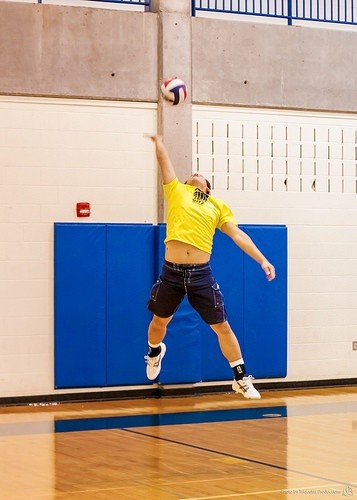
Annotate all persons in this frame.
[146,133,275,399]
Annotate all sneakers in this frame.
[232,376,261,399]
[146,342,166,380]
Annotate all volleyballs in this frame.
[160,78,188,105]
